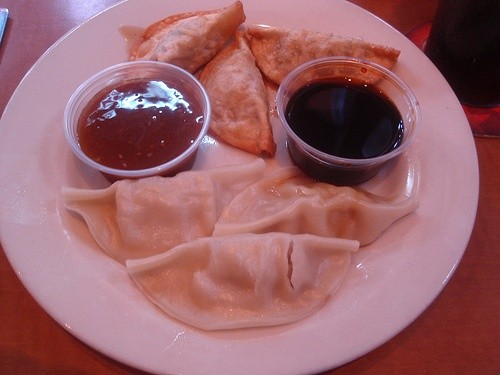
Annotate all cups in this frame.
[424,0,500,111]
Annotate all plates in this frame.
[0,0,480,375]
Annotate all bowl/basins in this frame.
[276,56,421,188]
[64,60,212,185]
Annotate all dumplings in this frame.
[61,158,420,333]
[127,0,401,157]
